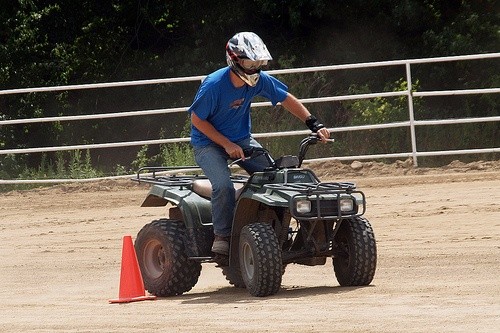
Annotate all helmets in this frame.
[226,32,273,88]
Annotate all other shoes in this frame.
[211,235,229,255]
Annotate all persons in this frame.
[187,32,332,257]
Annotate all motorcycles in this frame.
[130,132,377,298]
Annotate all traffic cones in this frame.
[109,236,157,303]
